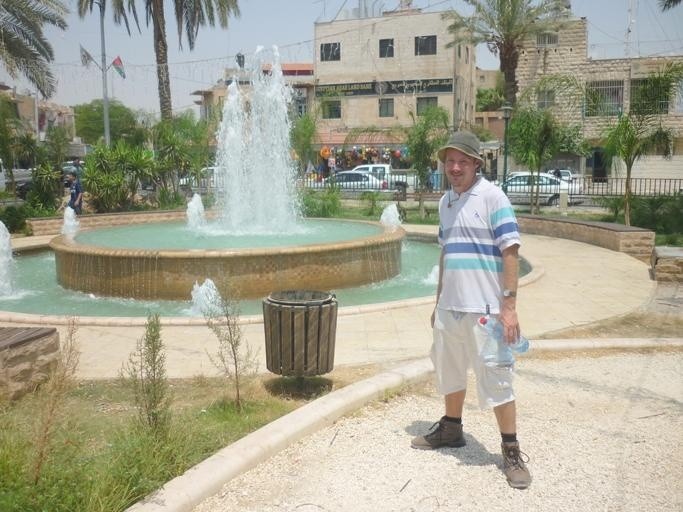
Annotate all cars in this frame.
[308,171,402,200]
[179,167,228,186]
[6,166,76,200]
[498,170,588,205]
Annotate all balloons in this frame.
[394,150,401,158]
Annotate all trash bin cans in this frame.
[262,290,337,397]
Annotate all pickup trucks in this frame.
[351,164,421,194]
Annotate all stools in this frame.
[0,324,61,410]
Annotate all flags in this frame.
[112,56,126,79]
[80,45,93,67]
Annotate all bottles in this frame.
[479,316,530,356]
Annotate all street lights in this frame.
[20,88,39,147]
[498,101,513,195]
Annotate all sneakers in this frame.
[410,416,466,450]
[501,440,532,490]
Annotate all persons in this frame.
[64,173,83,215]
[73,156,79,172]
[409,130,536,492]
[554,168,562,178]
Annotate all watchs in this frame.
[502,289,517,297]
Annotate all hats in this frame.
[437,129,486,171]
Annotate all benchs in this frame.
[513,211,655,258]
[24,208,215,236]
